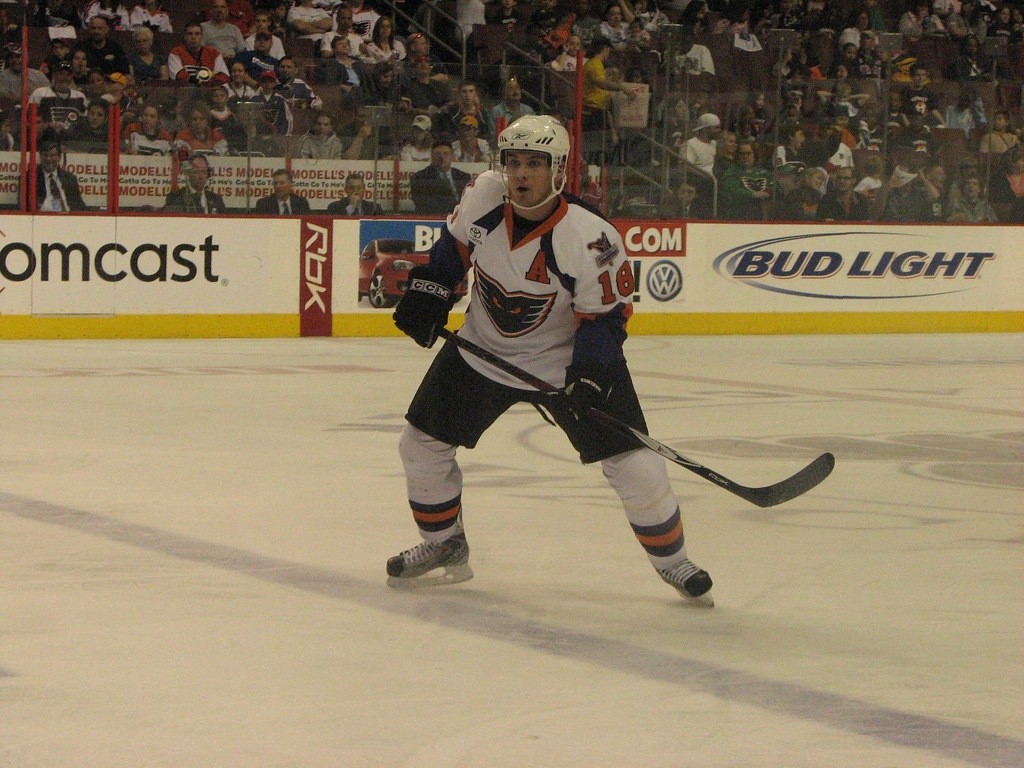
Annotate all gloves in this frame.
[393,267,456,349]
[547,324,629,424]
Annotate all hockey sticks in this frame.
[436,324,836,509]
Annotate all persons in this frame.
[456,0,1024,223]
[0,0,537,215]
[386,114,714,609]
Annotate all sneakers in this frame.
[386,505,473,589]
[658,559,716,609]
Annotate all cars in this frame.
[358,240,470,308]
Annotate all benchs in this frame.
[469,0,1024,124]
[27,0,434,156]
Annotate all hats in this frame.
[256,31,272,40]
[259,70,277,81]
[532,9,981,222]
[411,115,431,132]
[9,45,24,54]
[459,117,479,130]
[104,73,127,91]
[53,61,75,74]
[51,35,72,48]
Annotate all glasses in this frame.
[411,33,423,45]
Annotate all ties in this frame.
[283,202,291,216]
[48,173,66,212]
[442,173,452,191]
[354,208,360,217]
[192,192,205,214]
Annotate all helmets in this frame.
[498,114,570,174]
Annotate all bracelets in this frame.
[252,83,262,91]
